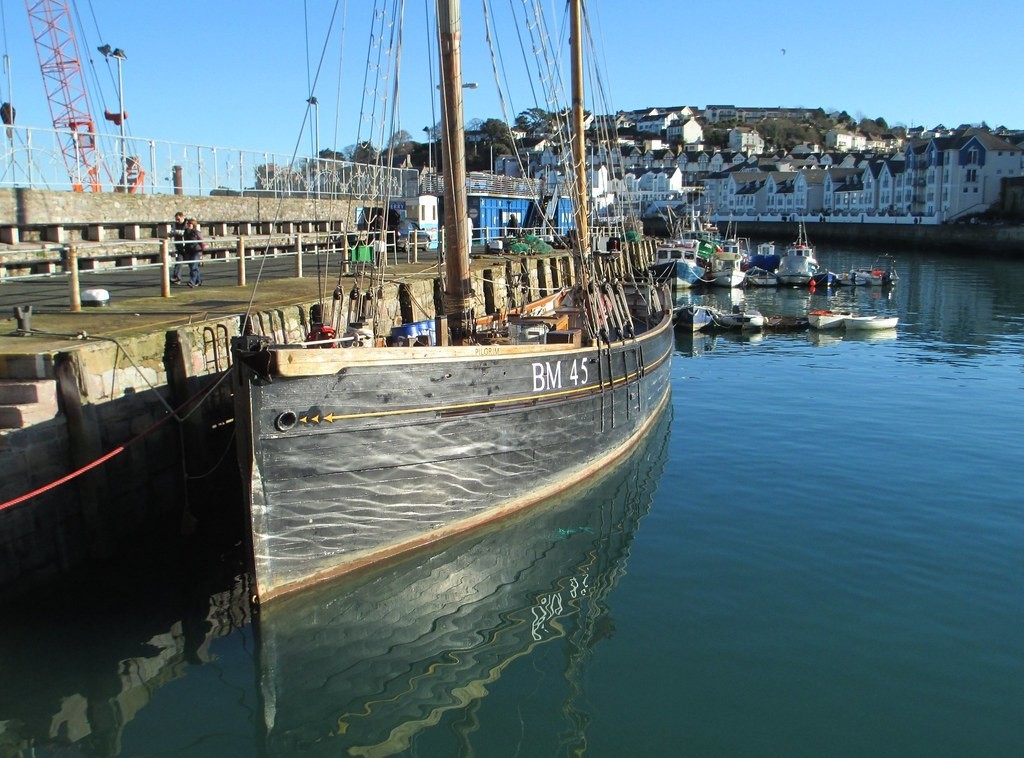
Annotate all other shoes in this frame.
[195,281,203,286]
[170,276,180,285]
[187,282,194,288]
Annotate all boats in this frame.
[772,215,821,285]
[675,304,718,332]
[839,265,900,286]
[844,315,900,330]
[644,181,777,288]
[806,309,849,329]
[811,268,840,287]
[767,313,810,327]
[717,309,764,329]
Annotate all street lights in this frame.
[97,43,130,193]
[306,97,321,200]
[421,126,432,195]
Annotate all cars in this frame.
[386,219,432,254]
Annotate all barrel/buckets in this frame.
[401,324,418,336]
[391,326,416,337]
[425,320,436,345]
[414,322,428,335]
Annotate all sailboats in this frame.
[227,0,678,605]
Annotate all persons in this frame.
[182,218,205,289]
[168,211,185,285]
[506,214,518,236]
